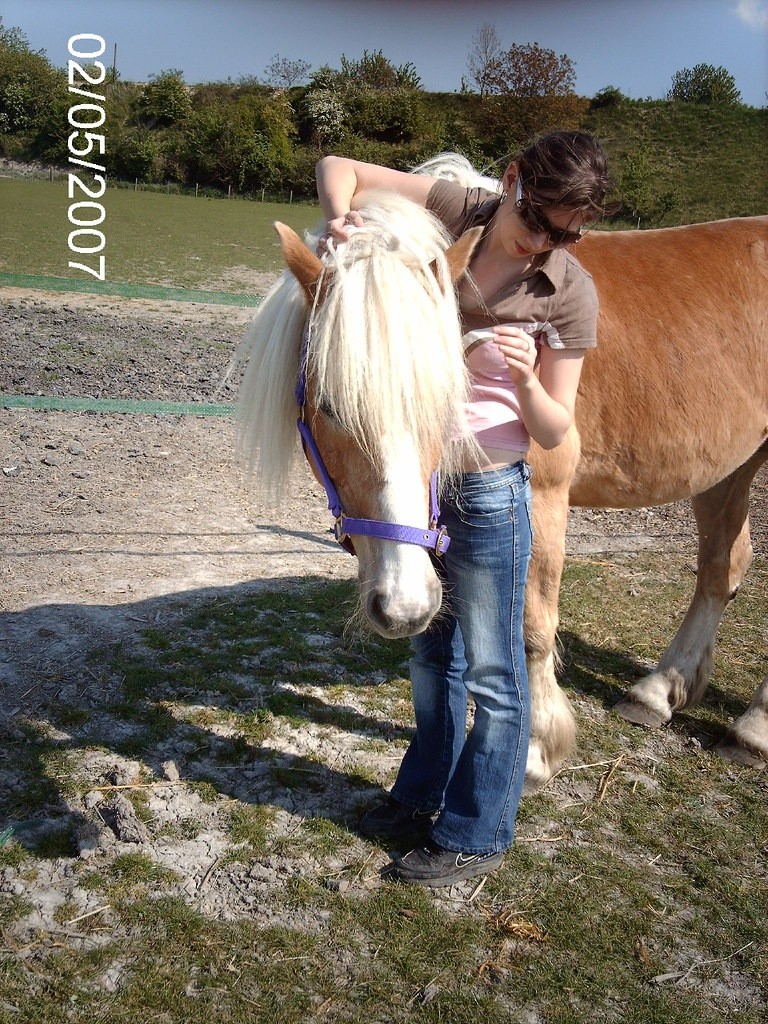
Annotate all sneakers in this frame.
[395,840,504,887]
[359,796,432,851]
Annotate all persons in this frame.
[316,132,608,887]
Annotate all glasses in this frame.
[515,173,583,250]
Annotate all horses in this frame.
[214,152,768,798]
